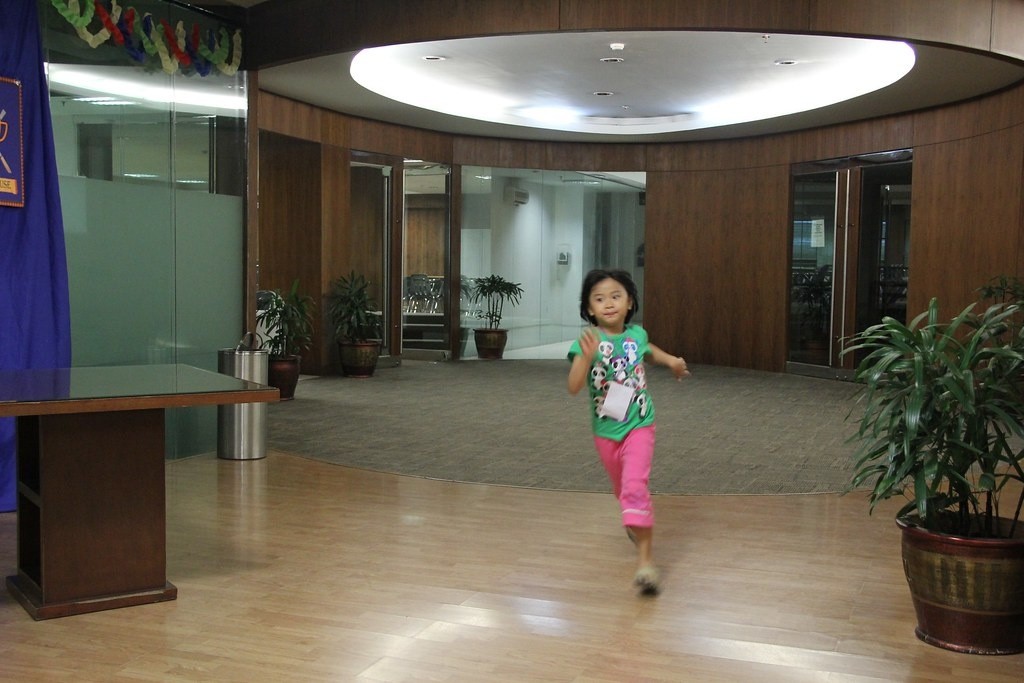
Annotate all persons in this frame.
[566,270,692,596]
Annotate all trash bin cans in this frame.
[256,291,285,353]
[218,332,270,461]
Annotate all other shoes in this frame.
[633,569,660,593]
[627,527,639,545]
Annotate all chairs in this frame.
[404,274,444,313]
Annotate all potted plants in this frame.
[835,296,1024,648]
[792,265,832,363]
[469,274,525,358]
[256,279,317,396]
[324,269,386,376]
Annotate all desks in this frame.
[428,276,444,280]
[0,362,283,624]
